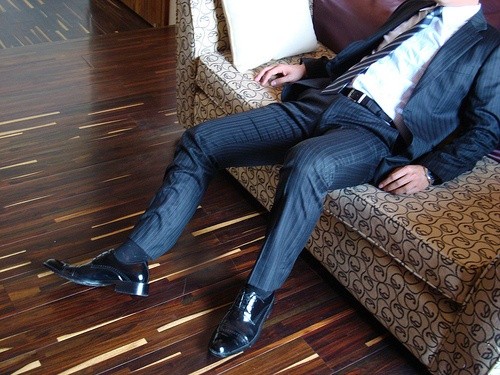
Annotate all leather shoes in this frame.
[43,248,149,297]
[207,285,276,358]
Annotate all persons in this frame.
[43,0,500,356]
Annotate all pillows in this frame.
[221,0,320,73]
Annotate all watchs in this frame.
[423,166,435,187]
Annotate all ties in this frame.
[320,6,444,94]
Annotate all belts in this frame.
[339,87,397,130]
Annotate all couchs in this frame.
[176,0,500,374]
